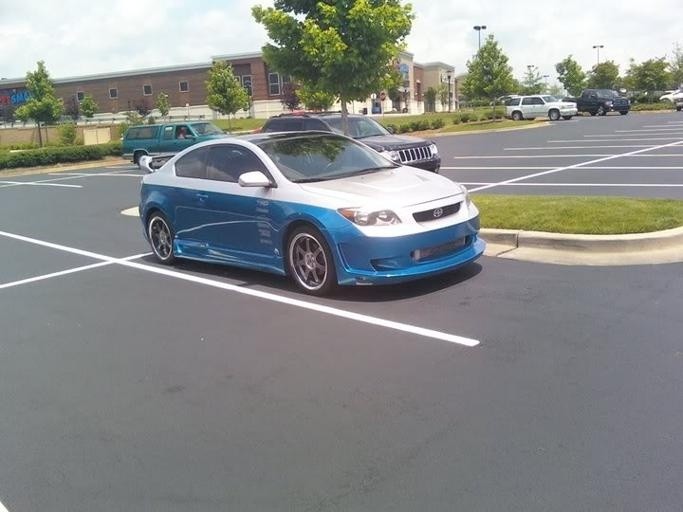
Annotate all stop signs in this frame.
[379,91,386,101]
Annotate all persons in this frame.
[177,127,188,139]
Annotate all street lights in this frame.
[528,65,534,89]
[543,75,549,94]
[473,25,486,49]
[447,70,452,112]
[593,46,603,63]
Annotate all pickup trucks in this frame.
[562,88,631,116]
[122,120,228,166]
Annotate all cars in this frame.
[659,83,683,111]
[41,114,77,126]
[489,94,578,121]
